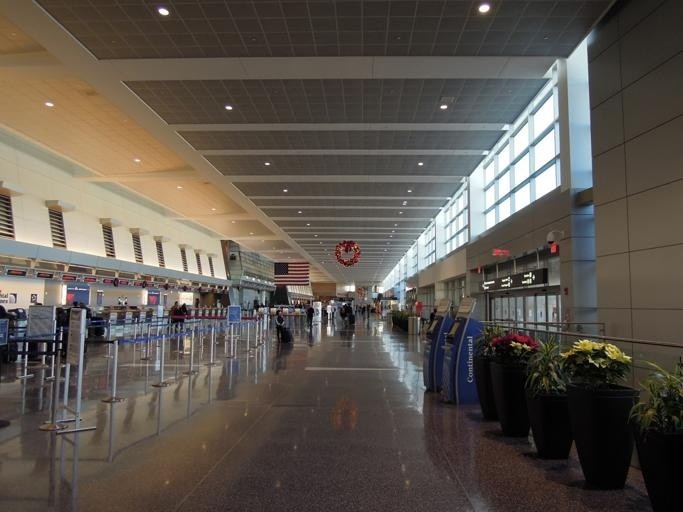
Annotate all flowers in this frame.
[472,318,682,444]
[335,240,361,267]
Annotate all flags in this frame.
[273,262,310,285]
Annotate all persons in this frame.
[174,303,187,329]
[307,305,315,323]
[340,303,370,322]
[167,300,178,324]
[275,309,287,344]
[79,302,91,318]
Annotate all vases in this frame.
[525,379,681,512]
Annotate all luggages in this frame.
[280,324,293,344]
